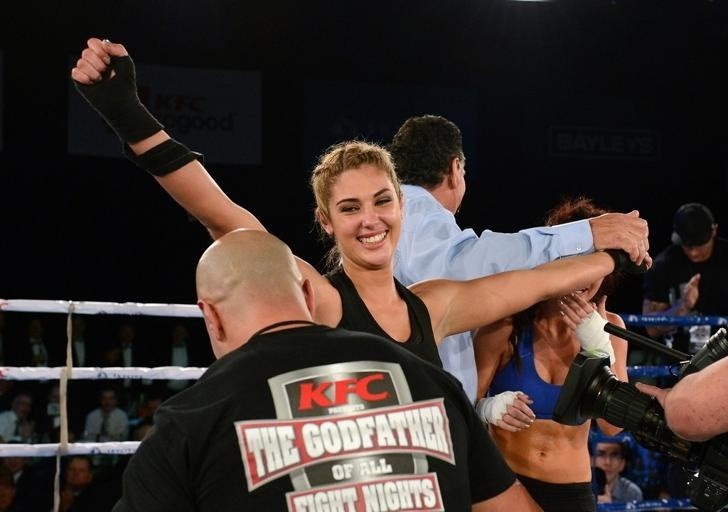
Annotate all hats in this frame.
[672,204,713,245]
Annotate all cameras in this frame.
[591,466,608,495]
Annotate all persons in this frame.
[391,115,649,404]
[70,36,652,369]
[590,440,689,505]
[643,204,728,353]
[636,356,728,440]
[472,202,629,511]
[2,314,196,511]
[112,227,543,512]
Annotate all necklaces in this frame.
[253,320,317,333]
